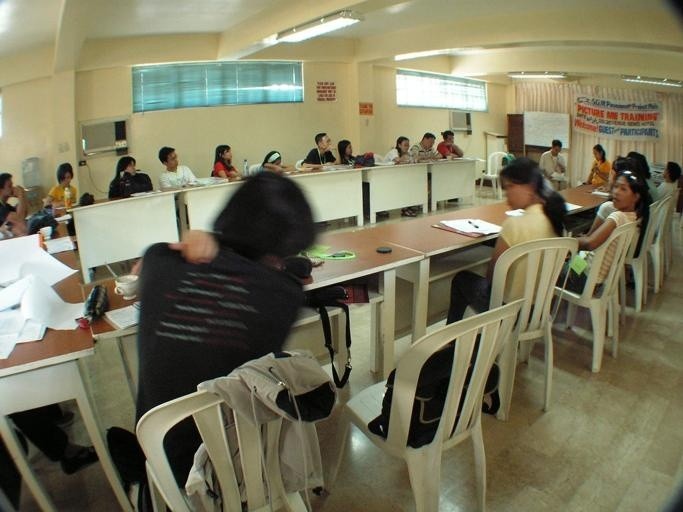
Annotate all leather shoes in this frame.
[54,410,75,428]
[60,444,99,476]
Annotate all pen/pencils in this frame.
[468,221,479,228]
[136,170,141,171]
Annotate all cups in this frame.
[114,275,137,302]
[446,156,452,161]
[37,226,52,241]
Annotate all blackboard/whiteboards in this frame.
[523,111,570,149]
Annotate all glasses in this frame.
[622,169,638,181]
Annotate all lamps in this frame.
[506,70,570,80]
[274,7,364,44]
[619,73,682,87]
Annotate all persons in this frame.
[261,151,293,173]
[110,147,197,197]
[214,145,240,177]
[0,403,100,477]
[384,131,463,216]
[301,132,356,167]
[109,171,313,511]
[539,140,680,296]
[0,162,78,223]
[445,158,568,346]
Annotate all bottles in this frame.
[119,180,130,198]
[77,284,108,329]
[63,187,72,211]
[242,159,248,178]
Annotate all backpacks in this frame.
[369,338,502,448]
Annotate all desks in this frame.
[66,157,476,284]
[91,181,613,422]
[0,218,134,512]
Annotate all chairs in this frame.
[625,187,683,315]
[459,235,581,413]
[133,352,331,512]
[554,220,642,374]
[330,297,529,512]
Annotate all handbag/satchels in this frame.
[305,286,350,307]
